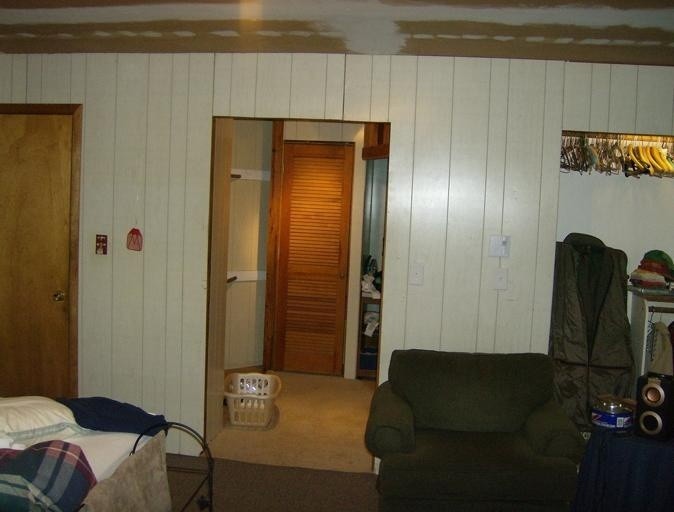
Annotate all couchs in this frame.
[363,349,585,512]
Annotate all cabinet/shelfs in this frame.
[356,296,382,378]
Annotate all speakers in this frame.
[635,371,673,443]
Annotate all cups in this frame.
[602,399,623,413]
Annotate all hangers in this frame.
[561,131,674,179]
[648,305,674,336]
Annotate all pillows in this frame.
[0,391,83,448]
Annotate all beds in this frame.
[0,395,168,512]
